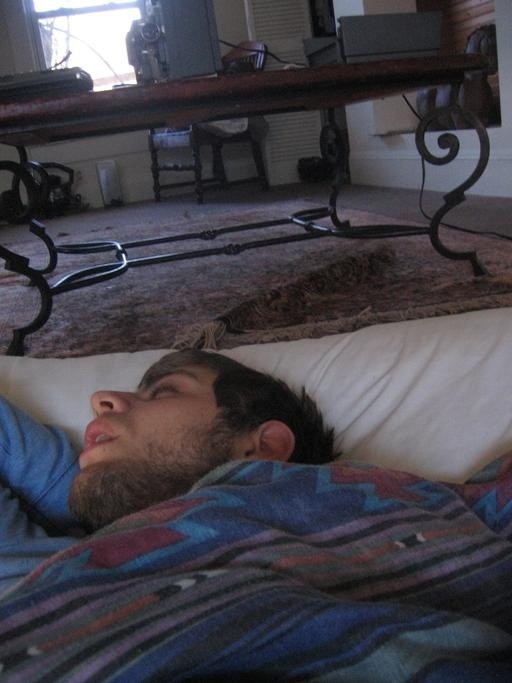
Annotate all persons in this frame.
[0,342,347,599]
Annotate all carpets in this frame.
[1,186,512,358]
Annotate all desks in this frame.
[1,22,500,358]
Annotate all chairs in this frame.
[146,40,271,205]
